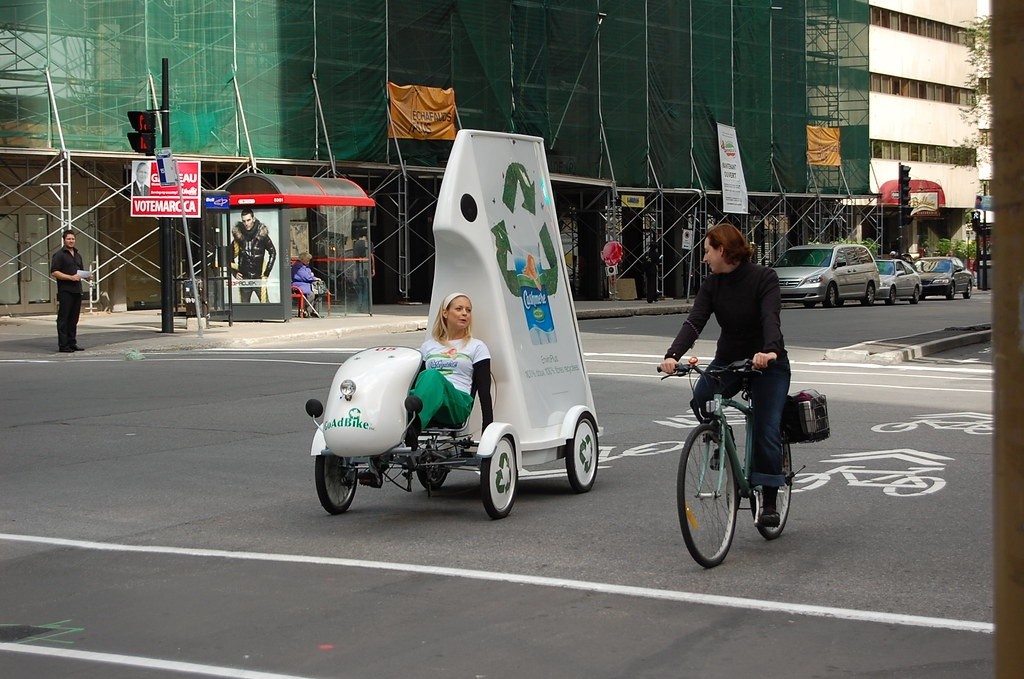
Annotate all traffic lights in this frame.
[899,164,911,204]
[971,211,980,230]
[902,206,914,226]
[126,109,157,156]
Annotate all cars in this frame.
[769,243,881,308]
[912,257,973,300]
[874,258,923,306]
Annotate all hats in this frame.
[648,241,660,247]
[889,251,896,255]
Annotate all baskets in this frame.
[788,388,831,444]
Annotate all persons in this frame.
[889,251,895,259]
[358,292,494,489]
[642,242,660,303]
[291,252,325,318]
[660,224,791,528]
[916,248,925,258]
[51,230,94,354]
[133,162,149,196]
[822,251,839,267]
[230,209,277,303]
[353,231,375,314]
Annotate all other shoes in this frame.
[357,467,384,488]
[311,311,326,318]
[69,343,85,351]
[757,513,781,525]
[709,442,737,470]
[58,346,75,352]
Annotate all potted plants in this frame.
[934,237,976,277]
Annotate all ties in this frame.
[140,185,144,196]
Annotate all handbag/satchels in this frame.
[312,280,328,295]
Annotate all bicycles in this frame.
[657,356,831,569]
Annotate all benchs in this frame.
[290,257,332,317]
